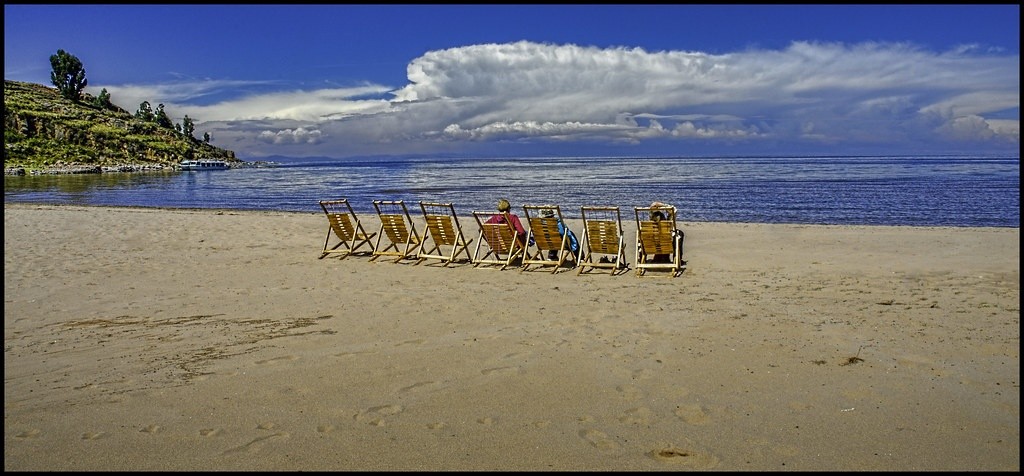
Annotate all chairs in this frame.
[367,199,429,265]
[575,205,626,275]
[411,201,473,267]
[317,199,377,260]
[633,205,682,279]
[521,204,577,274]
[471,210,531,271]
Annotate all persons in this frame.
[478,201,534,261]
[648,201,685,265]
[537,206,586,263]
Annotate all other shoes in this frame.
[612,256,623,266]
[547,255,558,261]
[600,256,610,263]
[576,257,584,263]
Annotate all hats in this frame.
[497,200,509,210]
[539,209,554,217]
